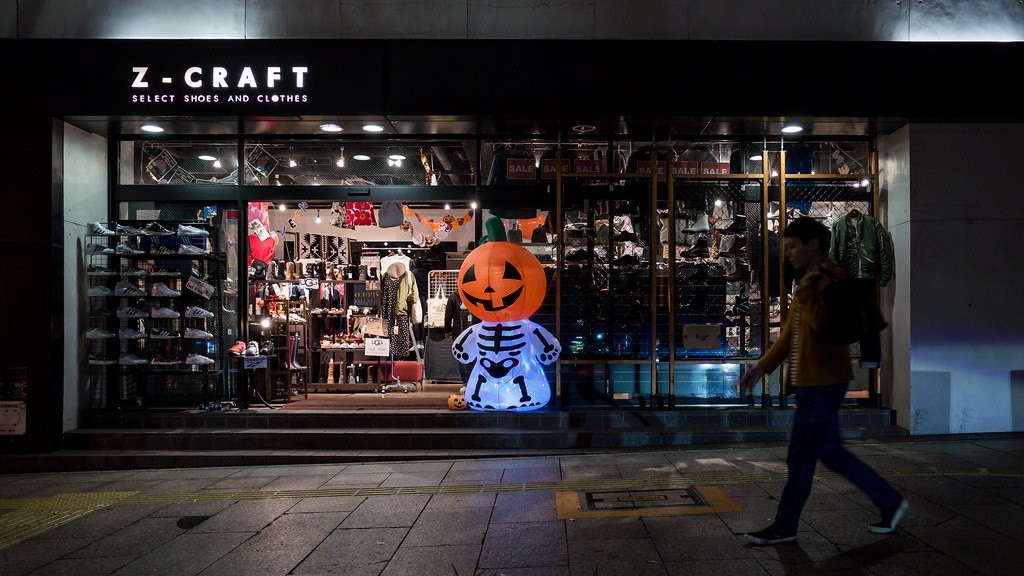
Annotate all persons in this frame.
[446,279,482,396]
[745,216,908,545]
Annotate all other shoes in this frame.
[866,499,909,534]
[555,196,757,337]
[743,524,796,543]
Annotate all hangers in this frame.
[848,201,860,217]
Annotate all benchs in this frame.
[327,361,423,391]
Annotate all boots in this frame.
[265,257,379,385]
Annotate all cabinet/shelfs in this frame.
[262,298,310,405]
[82,224,224,407]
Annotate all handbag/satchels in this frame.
[817,265,888,346]
[427,285,454,328]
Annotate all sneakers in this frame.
[245,341,259,355]
[228,341,247,355]
[261,341,275,355]
[85,218,222,369]
